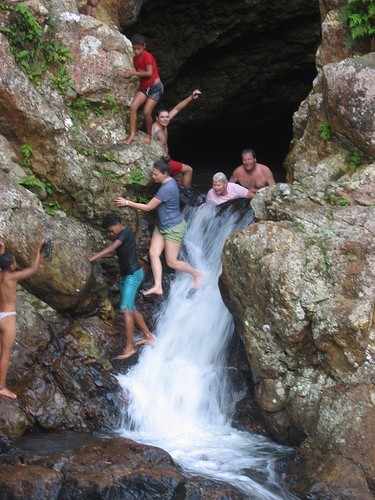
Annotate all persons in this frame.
[151,88,202,189]
[206,149,275,204]
[114,154,201,295]
[88,213,156,360]
[0,239,45,399]
[123,34,163,145]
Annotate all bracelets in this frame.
[125,199,128,206]
